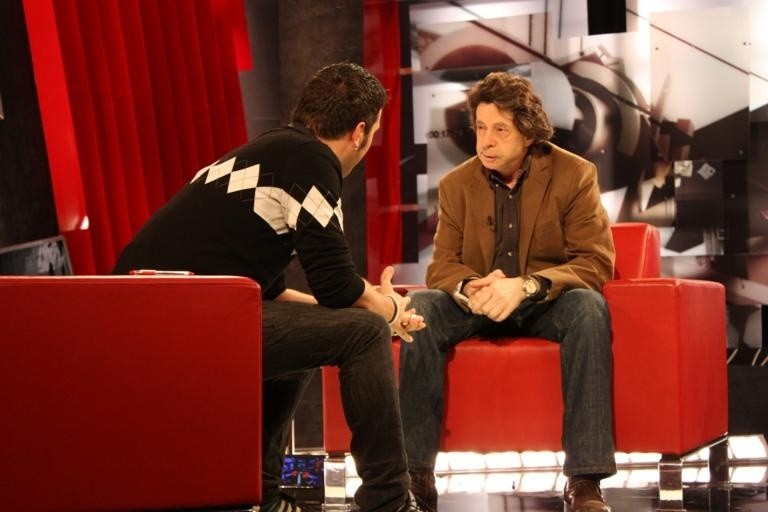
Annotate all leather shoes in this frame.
[563,476,610,512]
[409,479,438,512]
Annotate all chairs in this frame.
[319,221,735,512]
[0,273,265,512]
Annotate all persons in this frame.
[397,70,618,512]
[108,62,430,512]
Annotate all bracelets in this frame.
[387,295,400,324]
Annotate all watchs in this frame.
[520,274,538,298]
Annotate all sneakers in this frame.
[392,488,436,512]
[260,491,309,512]
